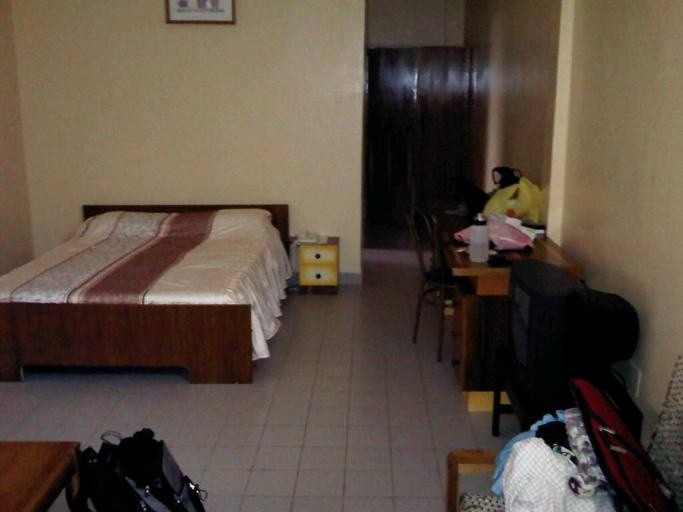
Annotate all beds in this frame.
[0,205,289,384]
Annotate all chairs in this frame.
[405,205,473,363]
[448,356,683,512]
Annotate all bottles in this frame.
[469,220,489,263]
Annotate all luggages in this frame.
[570,376,679,511]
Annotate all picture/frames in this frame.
[166,0,235,25]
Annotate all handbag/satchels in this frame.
[76,428,209,512]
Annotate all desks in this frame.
[0,441,81,512]
[443,235,582,387]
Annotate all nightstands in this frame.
[296,237,340,295]
